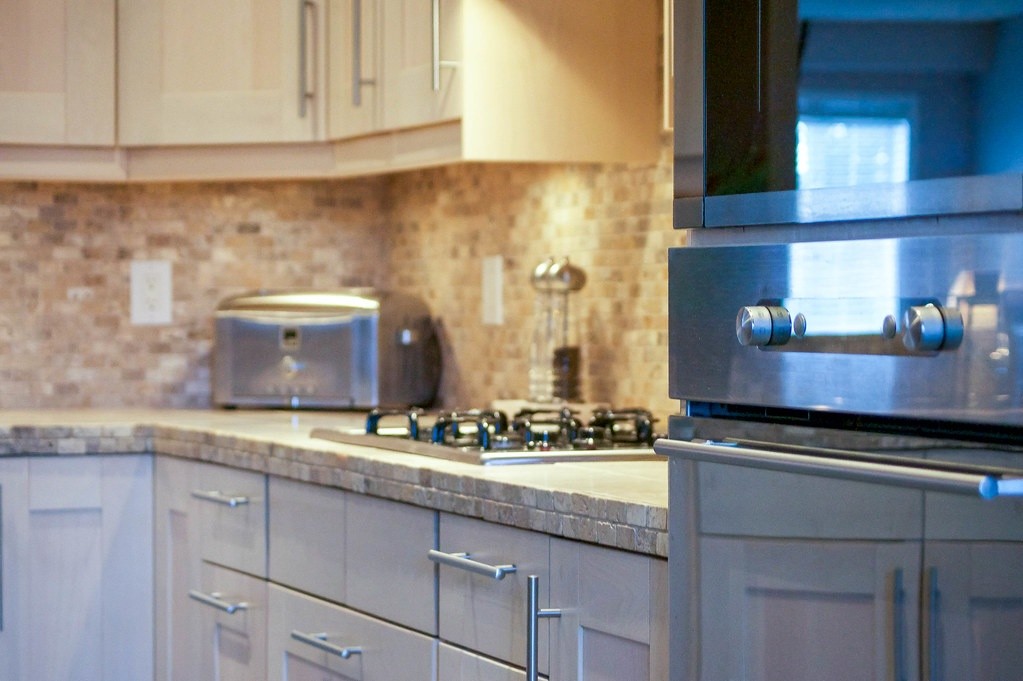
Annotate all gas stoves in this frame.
[308,394,667,467]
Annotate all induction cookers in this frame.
[205,288,439,415]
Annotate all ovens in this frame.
[666,3,1021,678]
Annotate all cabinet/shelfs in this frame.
[152,451,268,681]
[266,476,440,681]
[692,448,1023,681]
[0,0,128,184]
[326,0,663,181]
[116,0,325,184]
[426,512,670,681]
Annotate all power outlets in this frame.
[480,254,505,325]
[129,259,172,327]
